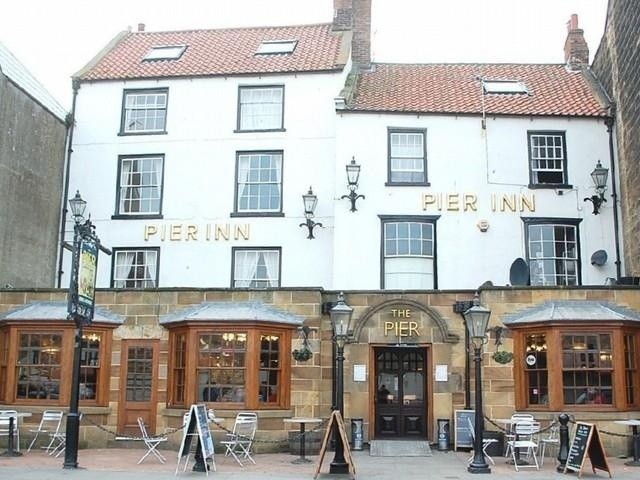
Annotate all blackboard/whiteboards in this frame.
[566,422,594,473]
[194,404,215,457]
[454,410,475,447]
[177,404,196,457]
[588,423,610,472]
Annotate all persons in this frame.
[258,381,272,402]
[14,368,47,399]
[378,384,390,404]
[576,387,598,404]
[203,379,219,402]
[591,386,608,405]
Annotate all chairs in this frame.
[220,413,259,467]
[136,417,168,464]
[1,408,83,457]
[467,412,561,469]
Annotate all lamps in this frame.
[341,154,365,213]
[584,159,609,215]
[299,185,323,239]
[68,189,87,226]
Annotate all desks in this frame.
[282,418,323,464]
[206,418,225,424]
[614,420,640,466]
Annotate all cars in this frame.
[17,346,98,399]
[198,359,278,402]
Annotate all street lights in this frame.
[328,292,355,474]
[463,291,492,473]
[62,190,100,470]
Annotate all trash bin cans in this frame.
[351,418,363,451]
[438,418,450,451]
